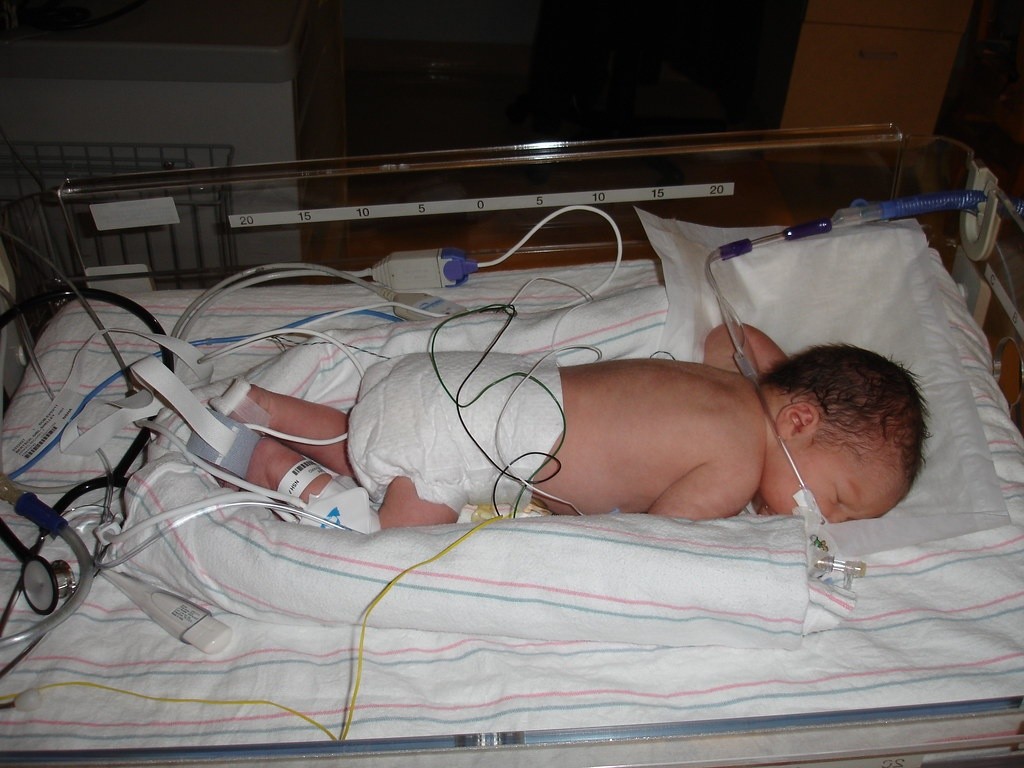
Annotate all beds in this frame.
[0,124,1024,767]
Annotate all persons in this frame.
[212,320,928,531]
